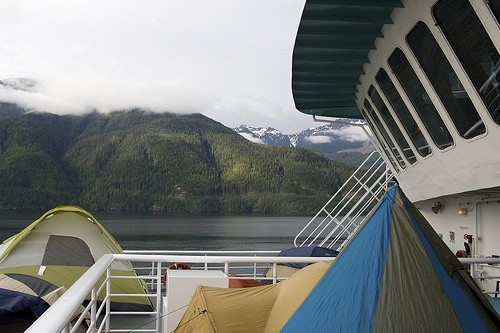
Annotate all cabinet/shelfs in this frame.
[162,268,229,333]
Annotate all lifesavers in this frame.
[165,264,192,276]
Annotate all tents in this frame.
[0,205,155,312]
[0,273,98,332]
[259,246,339,287]
[174,185,500,333]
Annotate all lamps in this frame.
[431,201,441,214]
[458,208,465,215]
